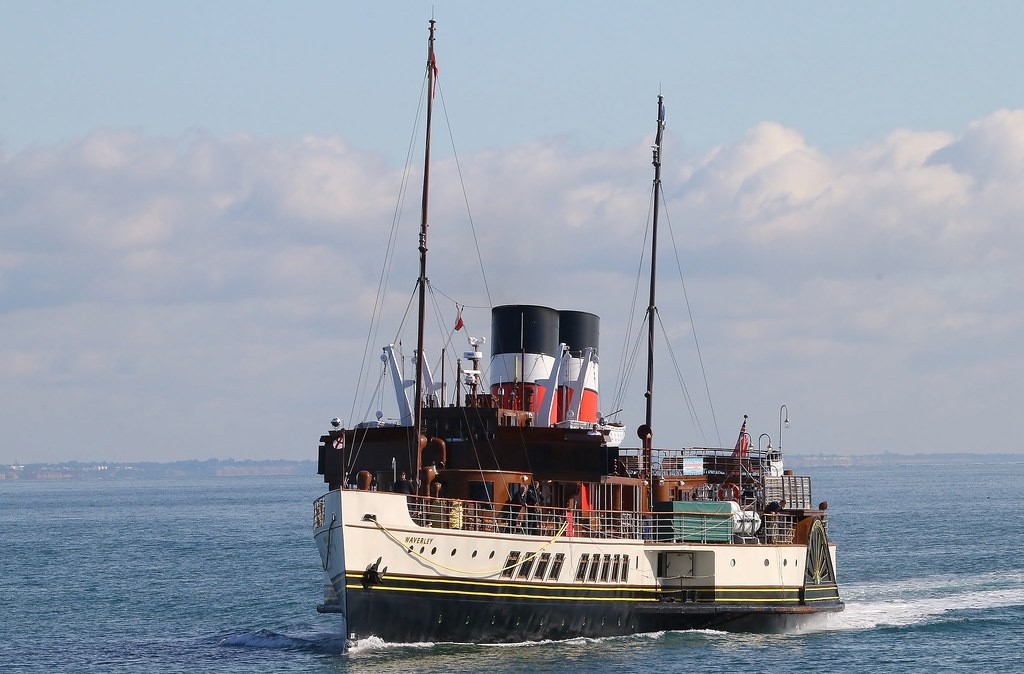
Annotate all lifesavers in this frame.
[718,482,740,503]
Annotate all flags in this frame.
[730,420,746,457]
[332,436,346,450]
[454,308,463,331]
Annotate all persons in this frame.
[504,480,539,535]
[394,470,415,517]
[765,499,786,544]
[587,424,601,436]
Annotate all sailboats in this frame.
[309,13,848,645]
